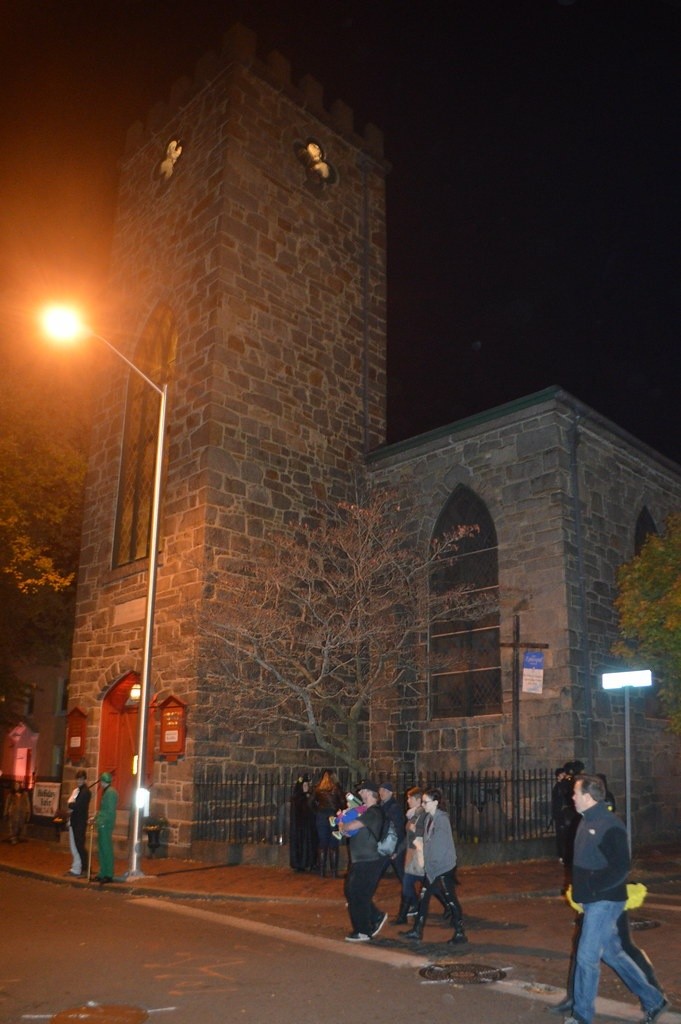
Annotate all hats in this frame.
[356,779,379,793]
[101,772,112,784]
[380,781,393,792]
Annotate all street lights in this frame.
[43,296,173,881]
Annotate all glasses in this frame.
[421,799,435,805]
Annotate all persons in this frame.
[551,774,672,1024]
[552,760,586,866]
[65,770,92,876]
[289,769,346,877]
[328,782,406,942]
[389,787,468,944]
[89,772,119,883]
[3,782,31,844]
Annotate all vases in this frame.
[147,830,160,849]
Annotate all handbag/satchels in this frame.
[412,814,424,869]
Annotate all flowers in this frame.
[141,815,167,832]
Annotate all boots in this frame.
[330,848,338,878]
[391,896,411,925]
[318,848,327,877]
[436,889,452,921]
[545,968,576,1014]
[400,915,426,940]
[448,920,468,945]
[634,948,665,996]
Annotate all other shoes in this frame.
[79,870,86,877]
[63,870,78,877]
[641,997,672,1024]
[332,831,342,839]
[564,1016,578,1024]
[91,877,100,882]
[407,905,418,915]
[329,816,336,827]
[101,876,111,883]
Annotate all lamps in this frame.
[130,683,141,703]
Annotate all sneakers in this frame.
[371,909,388,937]
[344,930,372,941]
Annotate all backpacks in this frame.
[366,806,398,856]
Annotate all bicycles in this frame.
[4,779,33,845]
